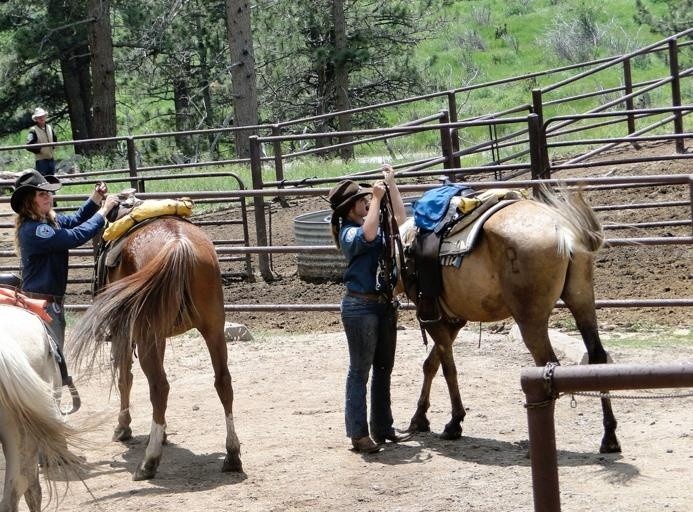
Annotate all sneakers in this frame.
[37,449,85,466]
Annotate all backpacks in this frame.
[414,184,471,231]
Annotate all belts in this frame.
[20,291,66,305]
[346,290,388,304]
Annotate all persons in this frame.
[8,167,121,469]
[326,162,419,454]
[25,108,57,210]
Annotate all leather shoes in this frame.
[371,430,412,444]
[352,435,380,452]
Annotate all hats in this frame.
[32,108,48,121]
[328,180,373,210]
[10,168,62,214]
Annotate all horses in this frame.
[62,194,243,482]
[1,302,134,511]
[388,175,622,454]
